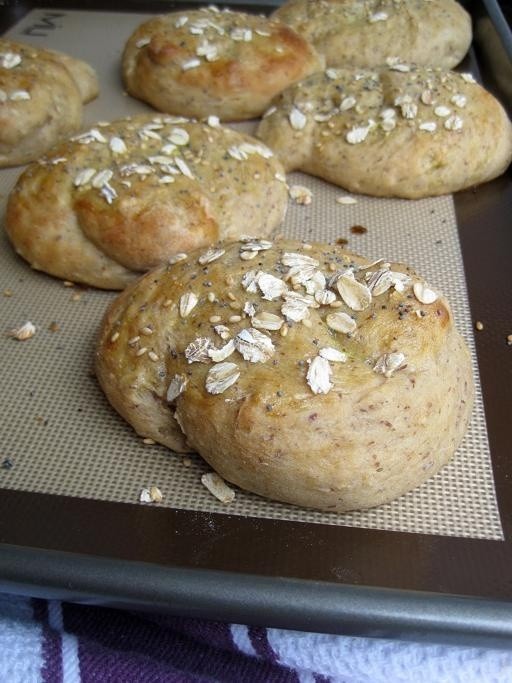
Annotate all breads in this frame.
[268,0,474,70]
[121,5,326,123]
[254,63,512,200]
[5,112,289,290]
[92,237,475,514]
[0,38,100,169]
